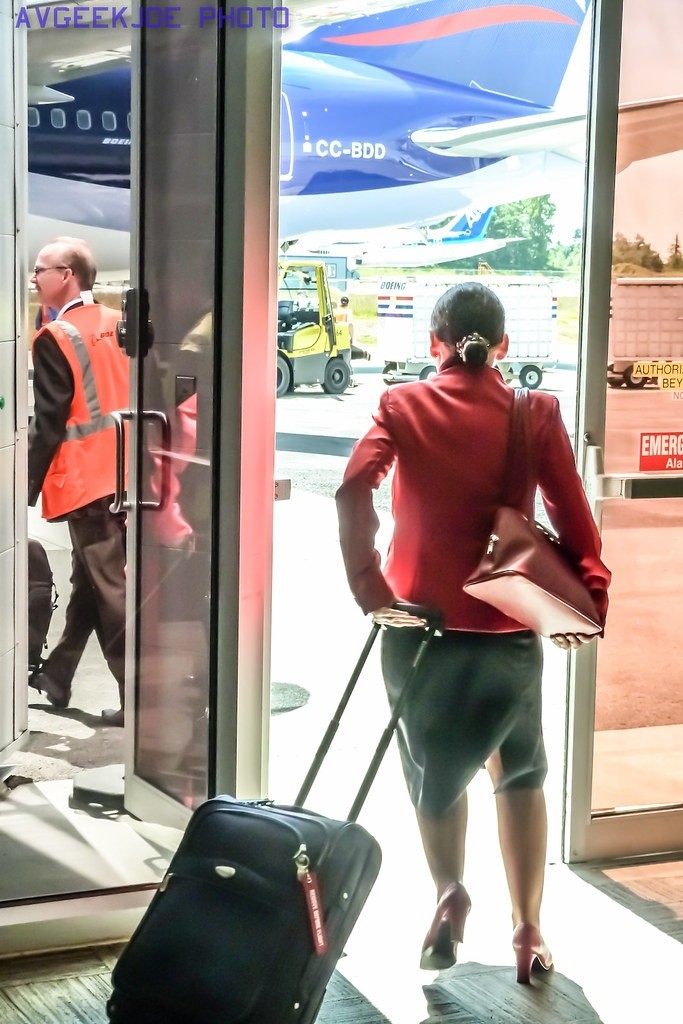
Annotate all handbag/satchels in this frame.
[462,389,603,639]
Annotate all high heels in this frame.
[512,923,552,984]
[420,881,471,970]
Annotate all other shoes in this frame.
[28,673,71,707]
[102,708,124,726]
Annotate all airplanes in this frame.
[30,0,682,270]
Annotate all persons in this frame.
[336,283,612,983]
[147,393,197,560]
[27,236,131,726]
[35,305,58,330]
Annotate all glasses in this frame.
[34,266,74,275]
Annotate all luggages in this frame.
[29,538,59,671]
[105,603,442,1024]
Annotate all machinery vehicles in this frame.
[607,360,674,389]
[277,261,351,396]
